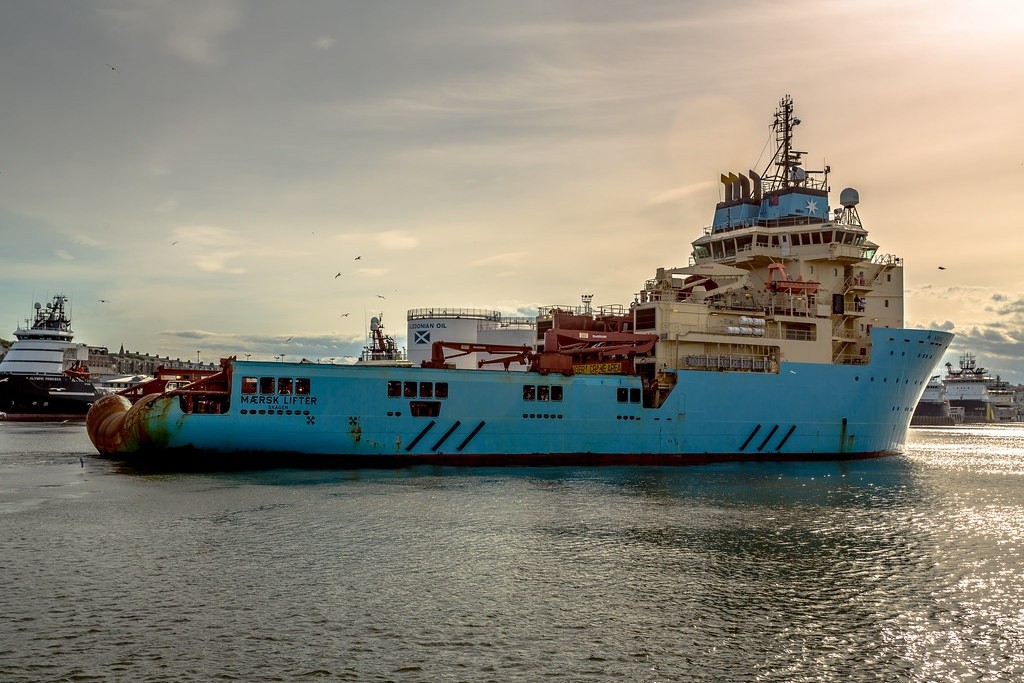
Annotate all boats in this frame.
[0,290,156,423]
[86,93,959,471]
[912,375,956,426]
[939,350,993,417]
[763,264,821,295]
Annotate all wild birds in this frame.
[97,299,110,304]
[171,241,178,245]
[340,312,351,317]
[938,266,946,270]
[286,337,293,342]
[375,294,385,299]
[335,272,342,278]
[50,387,65,392]
[355,256,362,260]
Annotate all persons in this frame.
[854,294,867,312]
